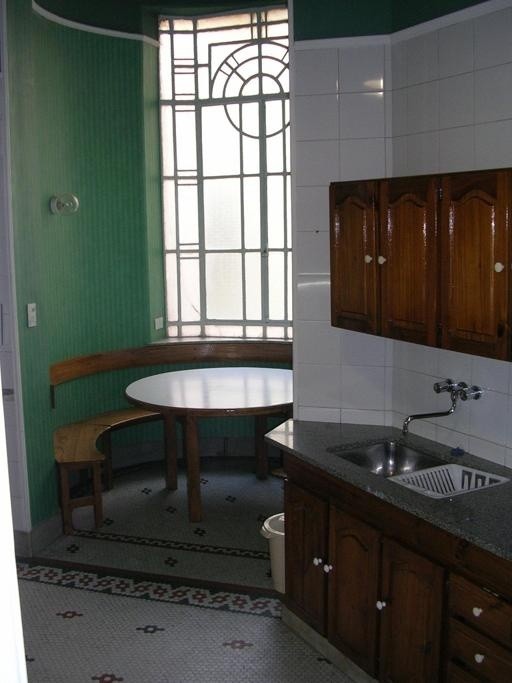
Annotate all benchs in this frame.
[49,334,293,532]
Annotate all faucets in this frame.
[401,384,464,433]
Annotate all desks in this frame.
[122,363,292,526]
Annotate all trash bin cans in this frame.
[260,512,286,595]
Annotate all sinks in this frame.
[331,438,450,479]
[391,464,508,499]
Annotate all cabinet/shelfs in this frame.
[380,536,446,682]
[281,480,378,671]
[327,179,434,346]
[445,572,511,679]
[434,168,511,356]
